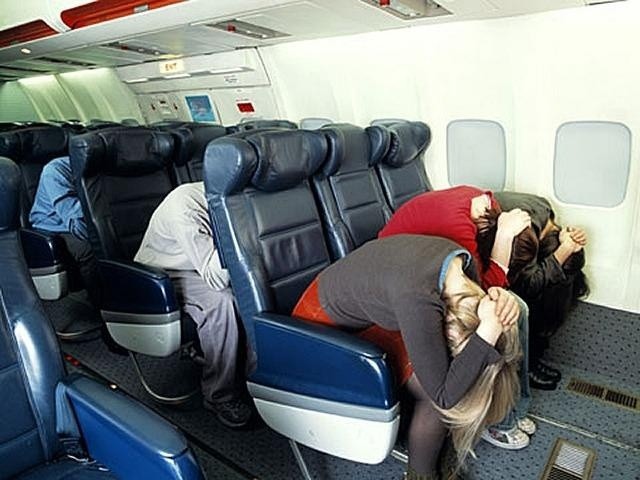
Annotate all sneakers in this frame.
[479,416,537,450]
[202,394,251,427]
[101,328,128,357]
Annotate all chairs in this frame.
[0,117,435,478]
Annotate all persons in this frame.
[133,181,253,429]
[28,156,101,275]
[291,233,523,480]
[492,190,589,390]
[380,185,540,450]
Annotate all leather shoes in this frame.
[530,361,561,390]
